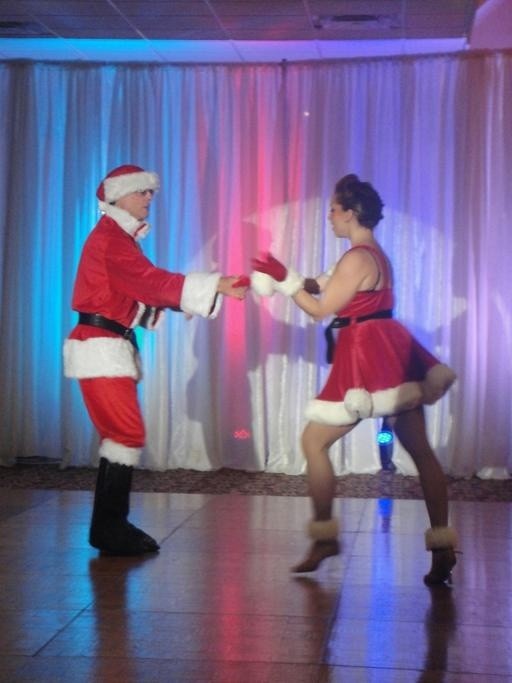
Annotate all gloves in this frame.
[233,271,275,297]
[251,253,305,298]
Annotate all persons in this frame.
[250,171,459,587]
[61,163,249,558]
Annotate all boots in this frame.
[290,520,342,572]
[423,526,463,586]
[88,438,160,554]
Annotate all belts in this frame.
[79,312,139,348]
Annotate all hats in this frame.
[97,163,160,205]
